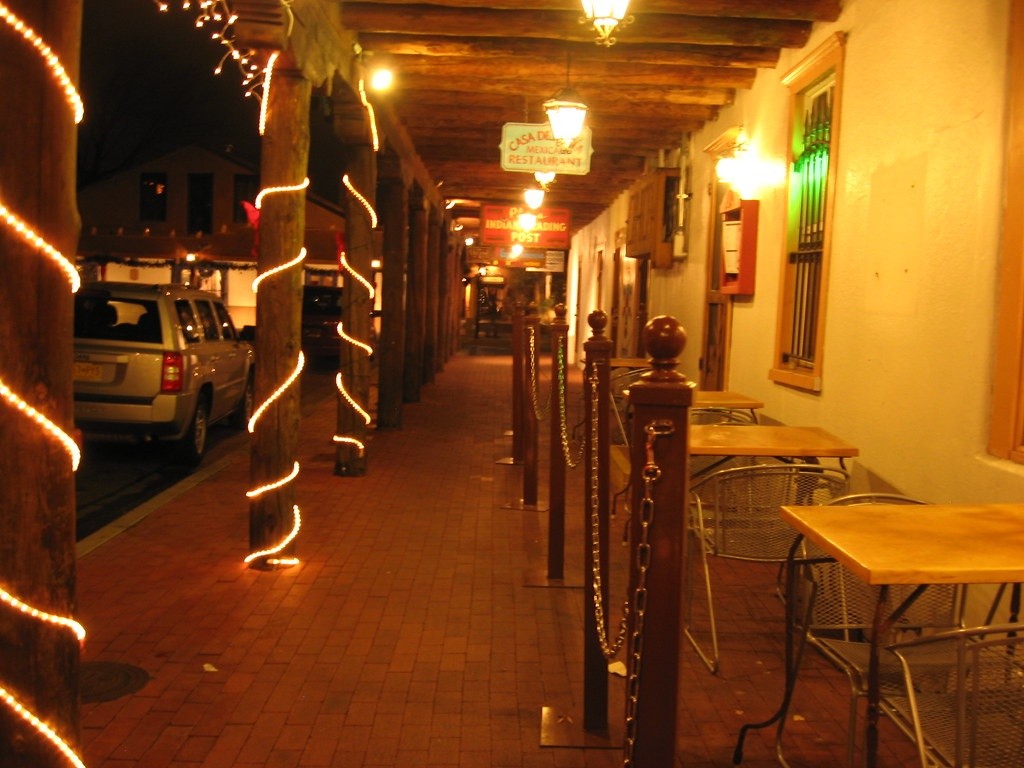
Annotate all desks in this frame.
[691,390,764,518]
[781,502,1024,768]
[688,423,860,624]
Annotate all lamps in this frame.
[578,0,634,49]
[542,43,589,150]
[532,172,557,193]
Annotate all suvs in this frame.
[302,285,345,362]
[71,280,258,468]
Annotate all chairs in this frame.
[579,370,1024,768]
[93,304,118,338]
